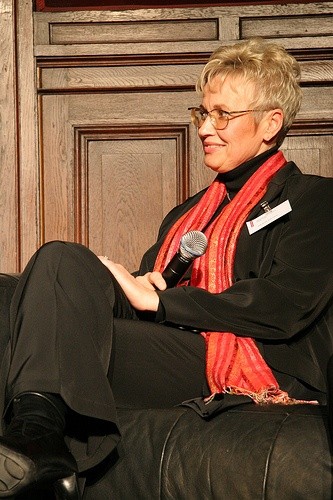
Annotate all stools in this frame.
[83,400,333,500]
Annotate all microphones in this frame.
[156,230,208,290]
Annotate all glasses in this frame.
[188,106,273,130]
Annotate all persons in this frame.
[0,36,333,500]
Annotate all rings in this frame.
[104,255,109,259]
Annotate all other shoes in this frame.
[0,392,77,498]
